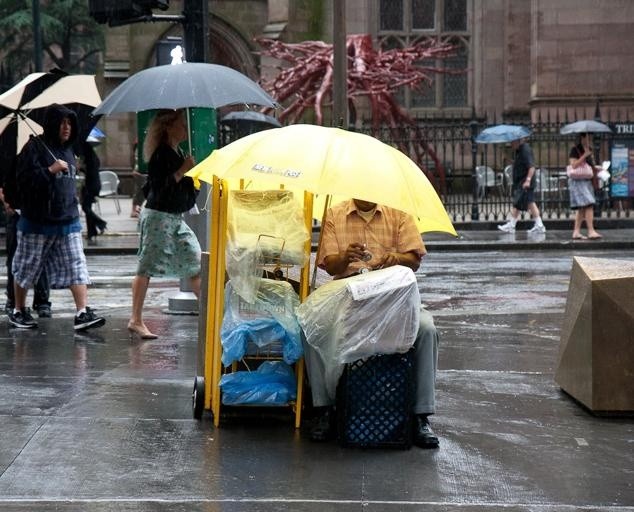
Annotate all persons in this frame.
[303,199,440,447]
[13,331,106,408]
[567,132,602,238]
[497,139,546,233]
[127,109,201,339]
[0,104,145,330]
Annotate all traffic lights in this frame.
[159,37,186,68]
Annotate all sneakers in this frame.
[497,224,516,235]
[7,311,38,329]
[526,226,546,234]
[73,305,106,331]
[571,231,603,239]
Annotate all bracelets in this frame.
[526,177,531,181]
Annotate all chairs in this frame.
[474,163,571,205]
[94,170,122,217]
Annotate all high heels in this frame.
[127,320,158,339]
[83,221,107,240]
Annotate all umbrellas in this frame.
[0,68,104,188]
[183,124,459,294]
[475,124,529,143]
[561,120,613,152]
[221,111,281,127]
[92,49,281,156]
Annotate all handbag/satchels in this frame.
[565,145,594,179]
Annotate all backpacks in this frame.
[3,133,41,210]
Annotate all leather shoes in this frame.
[414,416,440,450]
[37,304,52,318]
[309,411,339,443]
[4,300,33,322]
